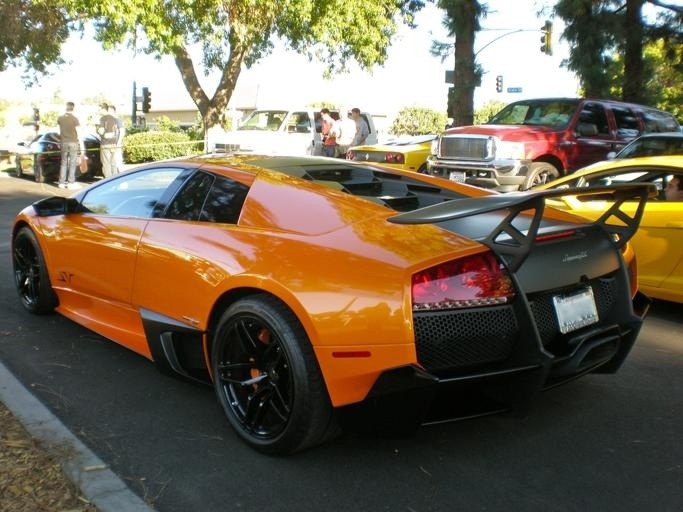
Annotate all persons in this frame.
[98,102,120,178]
[58,102,84,189]
[107,106,125,174]
[662,173,682,202]
[320,109,370,157]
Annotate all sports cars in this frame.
[345,134,435,173]
[8,153,656,456]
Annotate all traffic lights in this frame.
[33,107,40,121]
[539,20,553,56]
[142,87,151,113]
[495,75,502,92]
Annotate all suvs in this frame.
[427,97,679,193]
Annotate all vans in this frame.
[214,106,378,152]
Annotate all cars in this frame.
[14,133,103,181]
[521,155,682,304]
[598,132,682,191]
